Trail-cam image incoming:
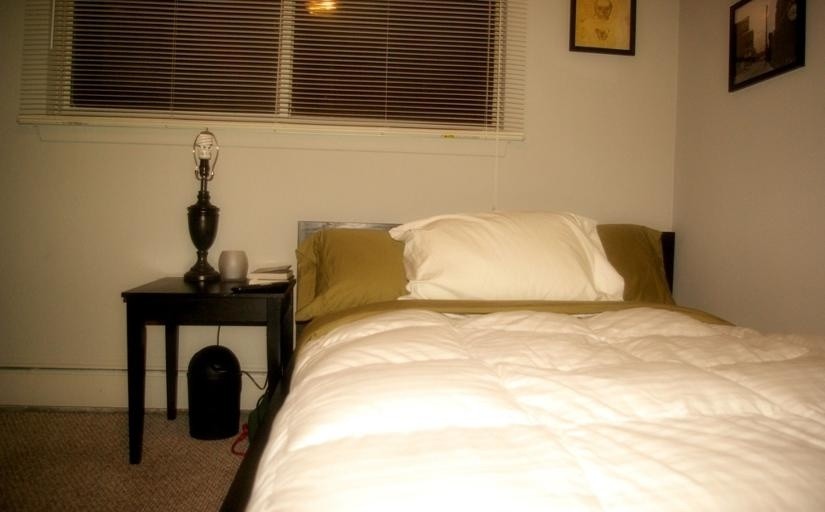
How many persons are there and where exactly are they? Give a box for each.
[579,0,629,49]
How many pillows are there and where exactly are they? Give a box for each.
[391,211,624,302]
[295,223,676,323]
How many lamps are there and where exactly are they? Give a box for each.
[181,128,221,284]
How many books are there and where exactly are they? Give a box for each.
[246,265,295,286]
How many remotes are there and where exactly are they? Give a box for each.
[231,282,290,294]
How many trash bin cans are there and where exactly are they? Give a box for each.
[186,344,243,442]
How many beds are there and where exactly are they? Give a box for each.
[219,220,825,512]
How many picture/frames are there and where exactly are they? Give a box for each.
[569,0,637,56]
[728,0,807,93]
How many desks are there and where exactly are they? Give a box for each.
[121,277,296,464]
